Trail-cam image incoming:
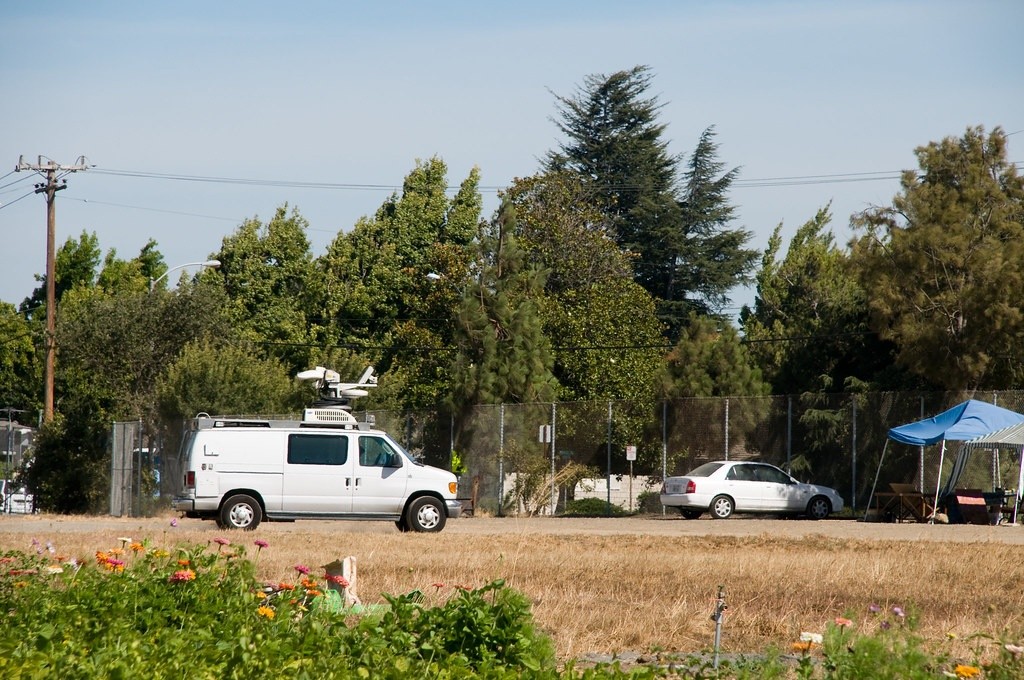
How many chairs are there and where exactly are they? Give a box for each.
[1001,495,1024,522]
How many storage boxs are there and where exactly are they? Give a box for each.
[944,489,991,524]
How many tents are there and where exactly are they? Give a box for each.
[863,400,1024,524]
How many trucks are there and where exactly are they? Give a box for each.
[1,477,36,513]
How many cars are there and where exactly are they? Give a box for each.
[659,461,845,521]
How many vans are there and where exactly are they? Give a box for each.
[172,406,461,533]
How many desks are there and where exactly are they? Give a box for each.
[874,493,936,524]
[983,492,1019,521]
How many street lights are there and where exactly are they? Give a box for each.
[134,259,222,516]
